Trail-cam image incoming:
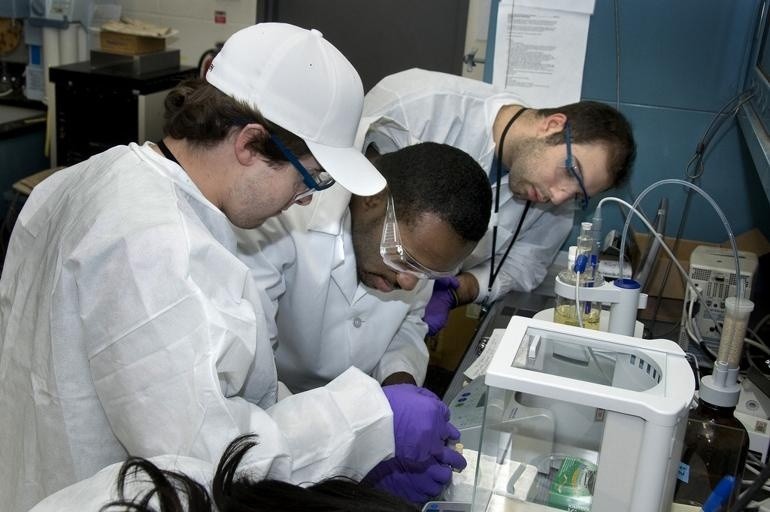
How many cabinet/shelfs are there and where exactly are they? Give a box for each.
[47,60,198,168]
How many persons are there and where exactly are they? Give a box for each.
[26,430,419,512]
[272,139,495,395]
[362,64,638,334]
[1,21,469,512]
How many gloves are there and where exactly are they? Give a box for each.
[365,429,465,503]
[380,382,454,470]
[422,277,459,334]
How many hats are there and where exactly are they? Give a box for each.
[203,19,388,198]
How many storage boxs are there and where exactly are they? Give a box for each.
[96,18,179,54]
[623,223,770,328]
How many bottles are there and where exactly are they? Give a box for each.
[672,375,750,510]
[554,221,605,330]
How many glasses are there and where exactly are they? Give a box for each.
[238,113,336,201]
[551,116,588,213]
[377,175,466,282]
